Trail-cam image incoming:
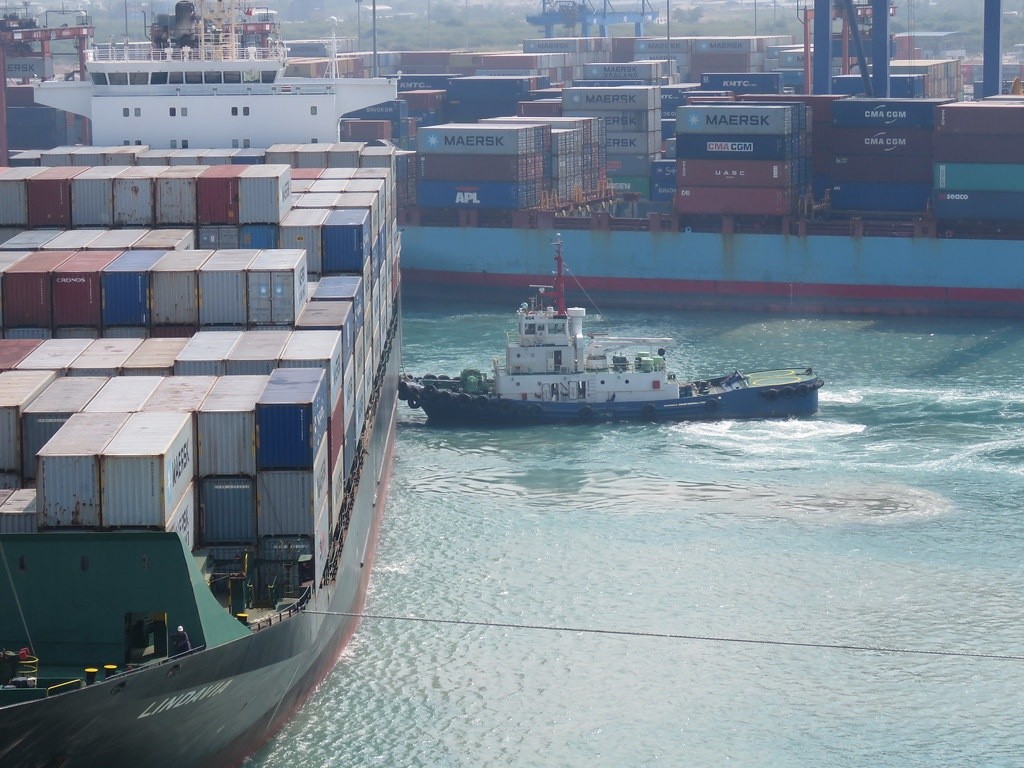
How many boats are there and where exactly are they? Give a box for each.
[397,232,823,430]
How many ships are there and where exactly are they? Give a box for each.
[0,156,397,767]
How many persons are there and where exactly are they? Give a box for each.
[172,626,191,651]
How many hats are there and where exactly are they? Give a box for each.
[177,625,183,632]
